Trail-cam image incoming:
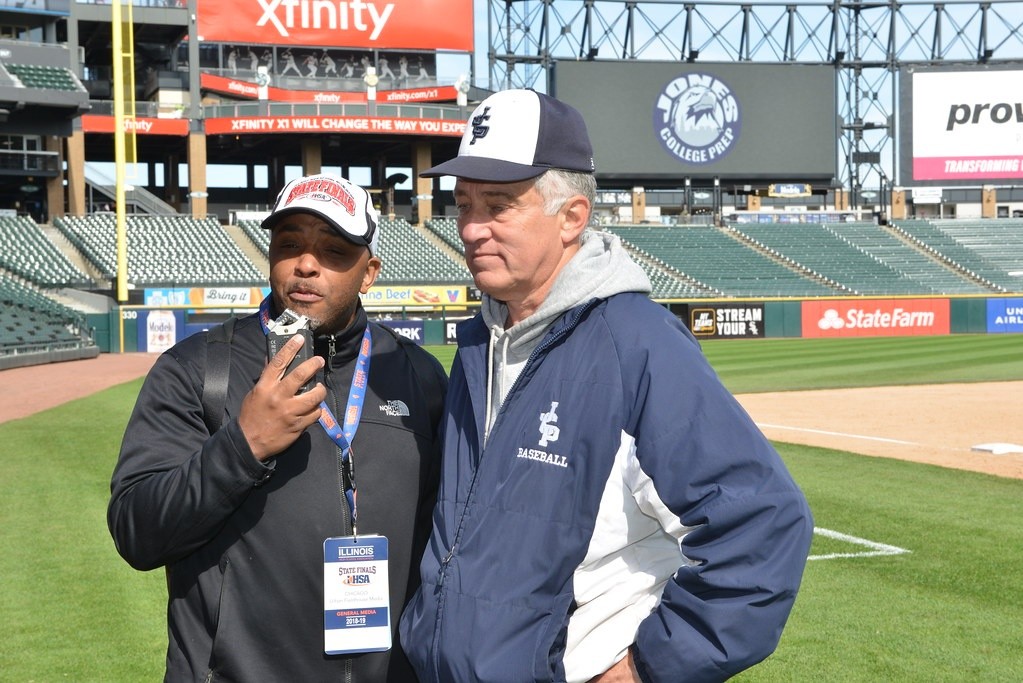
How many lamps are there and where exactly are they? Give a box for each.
[981,49,993,64]
[833,52,846,67]
[861,122,875,130]
[686,50,699,63]
[587,47,599,61]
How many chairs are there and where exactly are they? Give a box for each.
[0,61,82,92]
[0,213,476,352]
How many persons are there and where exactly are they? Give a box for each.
[227,49,429,82]
[107,176,449,683]
[397,87,814,683]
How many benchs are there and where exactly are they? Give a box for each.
[603,218,1023,299]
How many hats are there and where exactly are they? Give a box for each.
[261,173,380,257]
[418,87,597,182]
